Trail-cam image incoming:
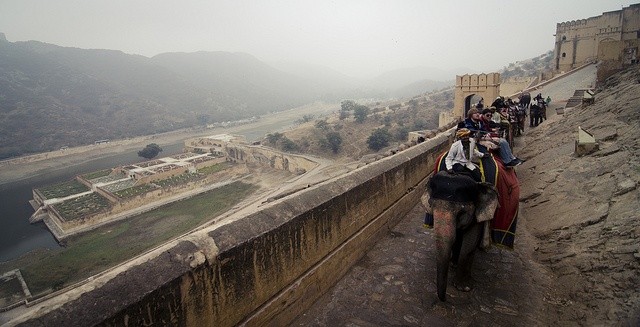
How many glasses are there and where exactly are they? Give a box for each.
[486,114,493,117]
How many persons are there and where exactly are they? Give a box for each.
[480,109,527,168]
[492,93,551,132]
[444,127,485,182]
[463,108,523,170]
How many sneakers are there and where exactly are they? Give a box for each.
[514,157,526,163]
[506,160,520,168]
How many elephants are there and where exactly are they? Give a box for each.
[529,103,543,127]
[517,92,531,109]
[420,147,520,303]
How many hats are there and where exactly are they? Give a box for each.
[456,127,471,138]
